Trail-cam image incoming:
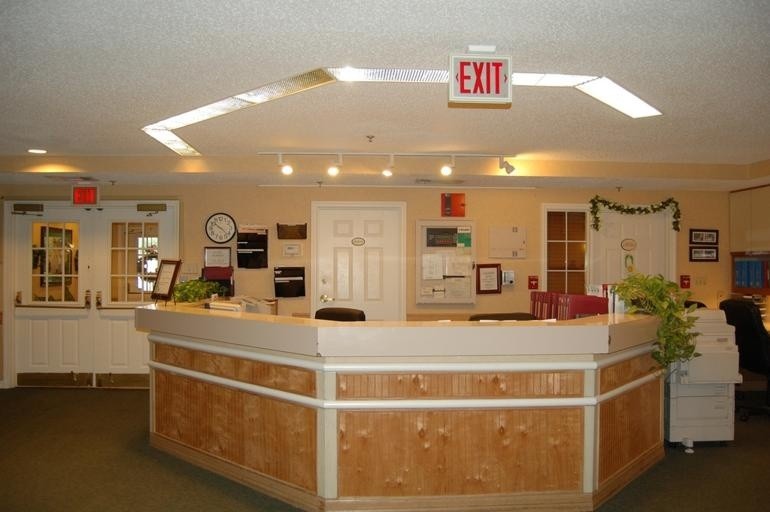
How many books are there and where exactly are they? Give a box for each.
[734,258,764,290]
[206,294,278,315]
[520,291,609,323]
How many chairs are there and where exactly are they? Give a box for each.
[720,298,770,374]
[316,307,366,320]
[469,312,537,321]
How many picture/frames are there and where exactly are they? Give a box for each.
[689,245,720,262]
[688,227,719,244]
[203,246,232,267]
[39,224,73,286]
[476,262,502,294]
[150,259,183,302]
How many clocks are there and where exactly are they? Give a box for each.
[205,212,236,244]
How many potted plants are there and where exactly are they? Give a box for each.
[610,273,702,370]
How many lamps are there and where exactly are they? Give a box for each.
[259,151,519,181]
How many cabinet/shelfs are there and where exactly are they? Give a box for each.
[663,309,743,454]
